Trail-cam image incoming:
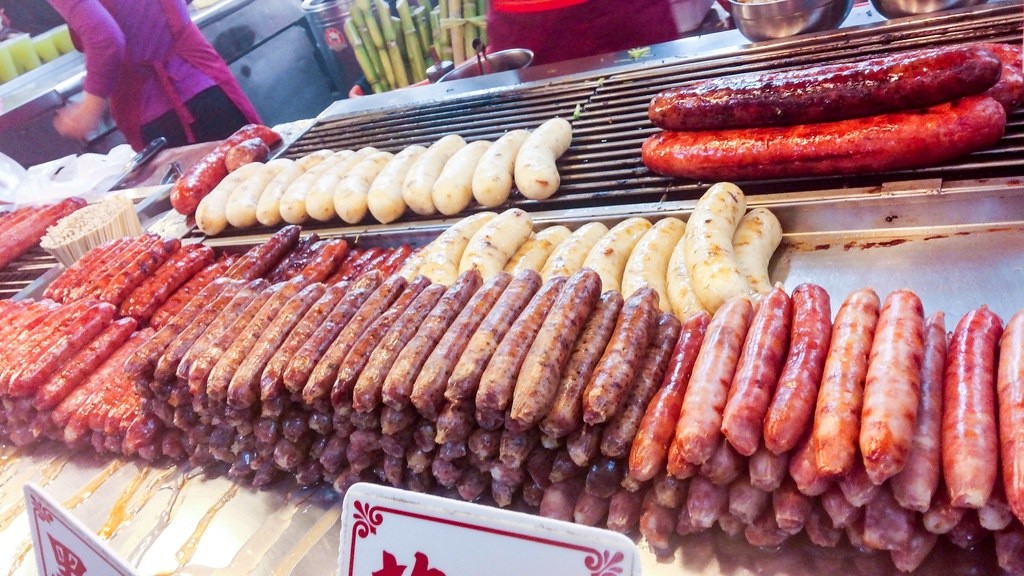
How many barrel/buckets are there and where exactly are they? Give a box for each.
[302,0,367,98]
[345,72,431,98]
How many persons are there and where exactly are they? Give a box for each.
[46,0,265,153]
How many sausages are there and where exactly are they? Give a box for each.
[0,41,1024,576]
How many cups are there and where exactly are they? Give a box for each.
[0,23,76,85]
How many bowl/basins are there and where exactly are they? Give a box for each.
[727,0,857,44]
[434,47,535,84]
[870,0,992,20]
